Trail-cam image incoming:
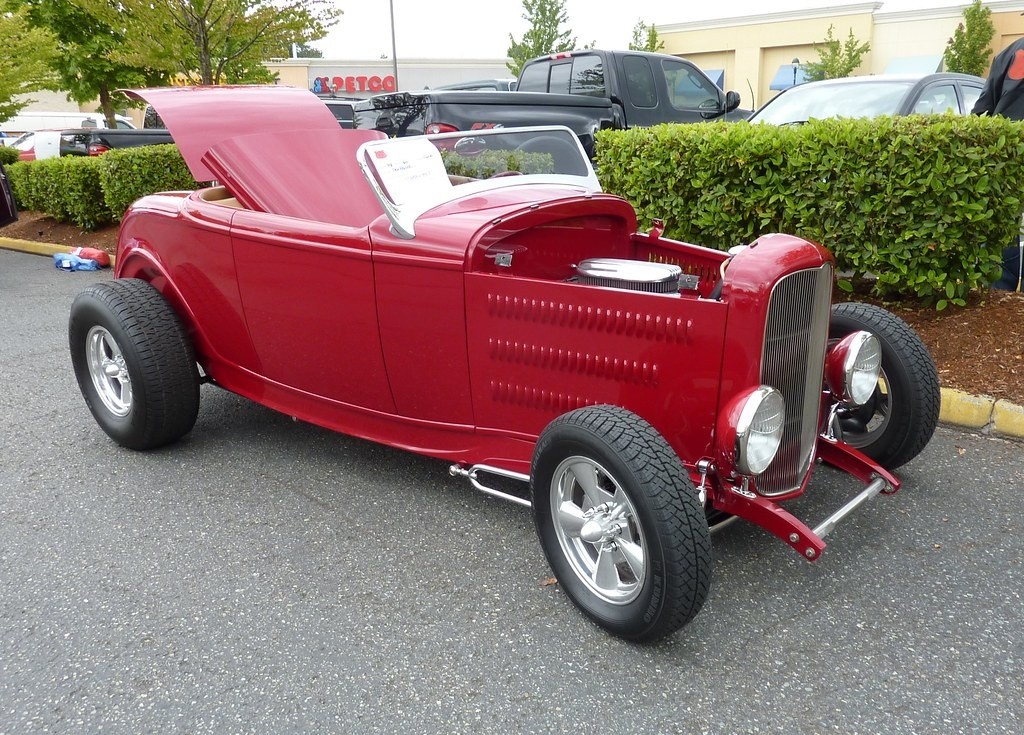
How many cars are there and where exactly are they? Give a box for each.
[10,129,60,161]
[741,72,984,125]
[65,85,942,644]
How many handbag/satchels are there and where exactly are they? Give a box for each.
[69,246,110,267]
[53,252,102,272]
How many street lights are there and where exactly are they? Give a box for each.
[791,59,799,84]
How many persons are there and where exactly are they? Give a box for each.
[970,13,1024,291]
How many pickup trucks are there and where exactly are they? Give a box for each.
[58,99,359,155]
[355,52,758,148]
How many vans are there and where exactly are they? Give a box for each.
[0,113,140,145]
